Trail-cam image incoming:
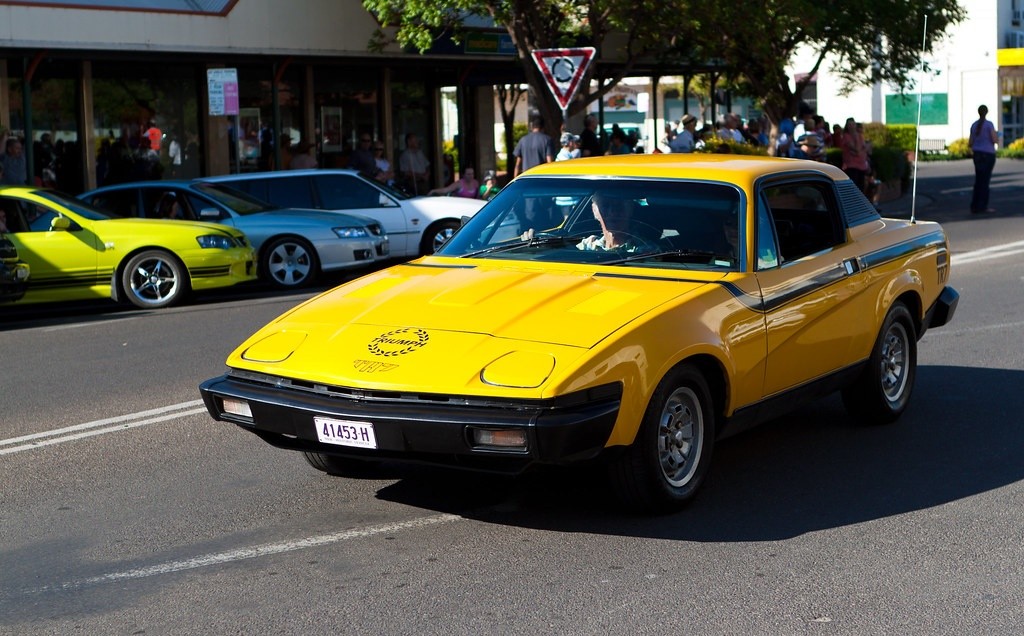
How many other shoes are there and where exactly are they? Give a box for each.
[972,208,996,216]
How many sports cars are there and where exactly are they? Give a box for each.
[200,151,961,516]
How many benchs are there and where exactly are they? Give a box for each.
[655,205,826,237]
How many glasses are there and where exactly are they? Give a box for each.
[361,140,371,143]
[373,148,383,152]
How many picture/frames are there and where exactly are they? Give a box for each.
[321,105,343,153]
[234,108,263,157]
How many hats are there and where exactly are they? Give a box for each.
[281,134,293,143]
[559,132,580,145]
[184,143,200,155]
[296,141,315,152]
[798,136,821,147]
[682,114,698,128]
[482,170,498,181]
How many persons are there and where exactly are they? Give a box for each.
[554,113,882,215]
[0,208,6,233]
[479,169,500,201]
[157,194,179,219]
[512,116,552,178]
[257,129,434,196]
[521,187,637,253]
[427,166,480,199]
[0,119,182,197]
[968,105,999,213]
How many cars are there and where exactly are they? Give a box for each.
[401,195,572,261]
[208,168,427,265]
[1,186,258,312]
[27,180,391,290]
